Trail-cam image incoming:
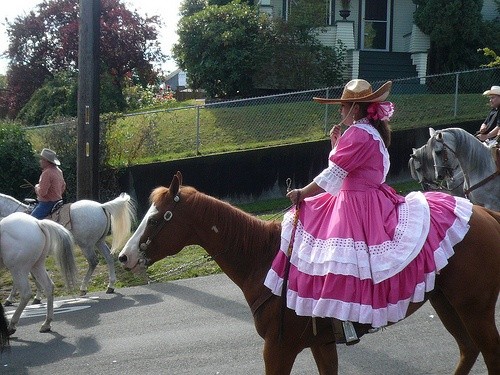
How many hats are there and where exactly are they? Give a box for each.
[483,86,500,95]
[312,78,392,126]
[33,148,61,165]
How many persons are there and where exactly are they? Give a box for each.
[263,78,474,328]
[476,86,500,149]
[28,147,67,219]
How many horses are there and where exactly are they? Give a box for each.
[119,170,500,375]
[408,127,500,211]
[0,192,136,355]
